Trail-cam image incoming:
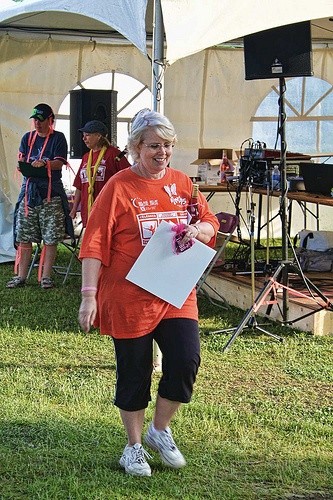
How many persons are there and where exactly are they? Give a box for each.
[6,103,76,288]
[78,109,220,477]
[68,120,131,228]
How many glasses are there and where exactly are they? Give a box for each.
[140,142,174,149]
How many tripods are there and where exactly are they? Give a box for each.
[202,77,333,354]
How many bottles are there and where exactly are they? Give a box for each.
[221,149,229,182]
[187,183,202,225]
[71,146,74,157]
[271,165,280,189]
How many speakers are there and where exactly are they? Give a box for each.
[69,89,118,160]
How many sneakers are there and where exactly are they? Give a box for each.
[145,425,186,468]
[119,441,153,477]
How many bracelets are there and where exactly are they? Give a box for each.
[191,224,200,236]
[81,287,97,292]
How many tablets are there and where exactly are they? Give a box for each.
[17,160,49,177]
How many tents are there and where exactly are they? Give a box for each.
[0,0,333,264]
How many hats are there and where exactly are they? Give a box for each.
[28,104,53,121]
[78,120,108,138]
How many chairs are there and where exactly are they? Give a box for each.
[27,196,84,284]
[196,213,238,312]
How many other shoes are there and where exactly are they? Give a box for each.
[6,278,25,288]
[42,277,53,288]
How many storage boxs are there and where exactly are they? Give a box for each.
[208,232,227,267]
[190,148,233,186]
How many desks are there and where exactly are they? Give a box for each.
[199,185,333,269]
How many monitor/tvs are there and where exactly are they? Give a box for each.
[298,161,333,197]
[243,20,314,80]
